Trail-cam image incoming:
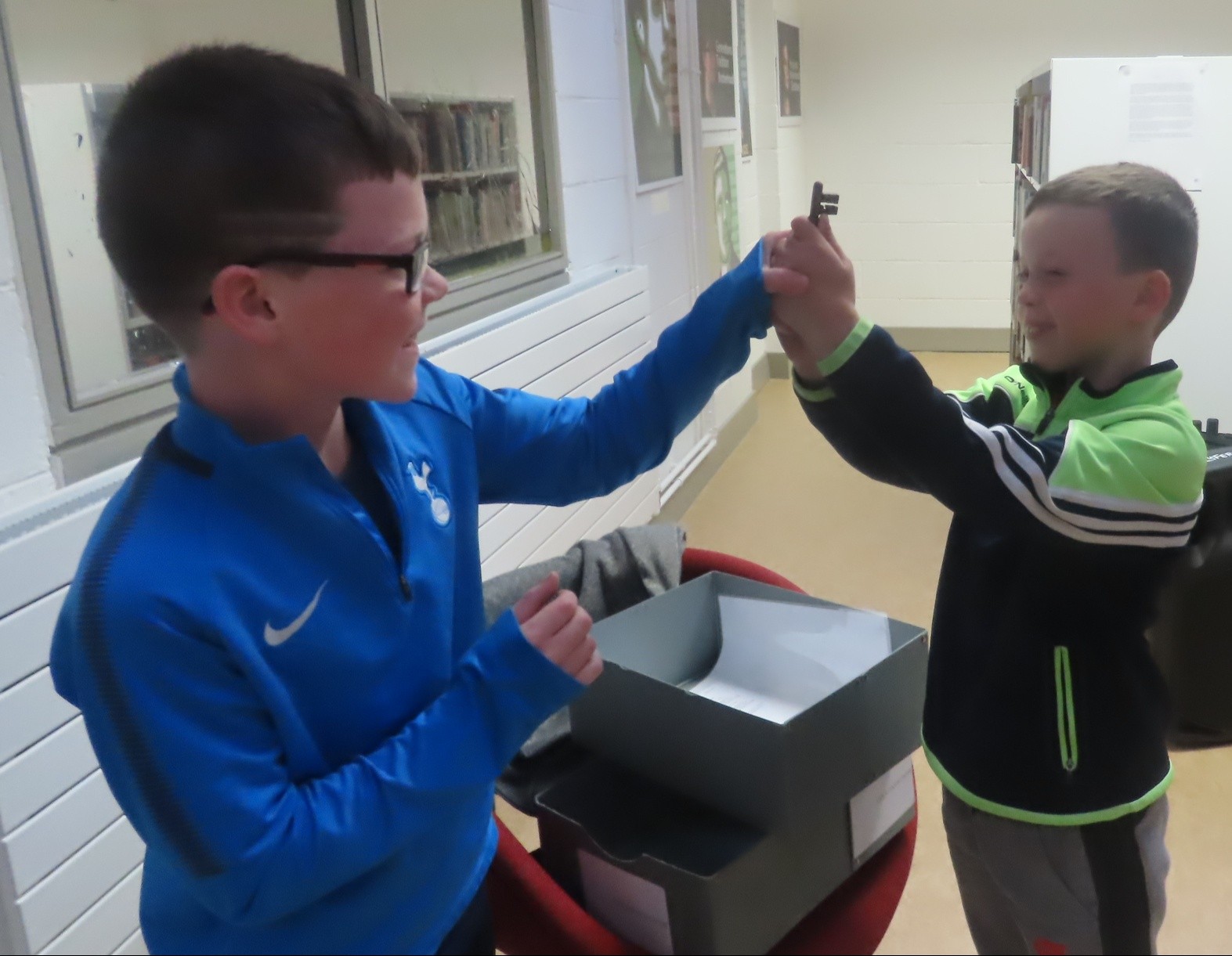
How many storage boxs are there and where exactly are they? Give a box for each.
[535,757,918,956]
[566,570,930,832]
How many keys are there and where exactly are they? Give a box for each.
[807,180,839,225]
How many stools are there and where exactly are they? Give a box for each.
[448,546,917,956]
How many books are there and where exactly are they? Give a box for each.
[1010,96,1050,366]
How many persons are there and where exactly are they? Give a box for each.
[768,163,1205,956]
[51,40,811,956]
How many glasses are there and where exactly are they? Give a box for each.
[197,239,434,317]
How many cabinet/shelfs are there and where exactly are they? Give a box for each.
[393,97,527,271]
[1010,56,1232,432]
[16,81,173,395]
